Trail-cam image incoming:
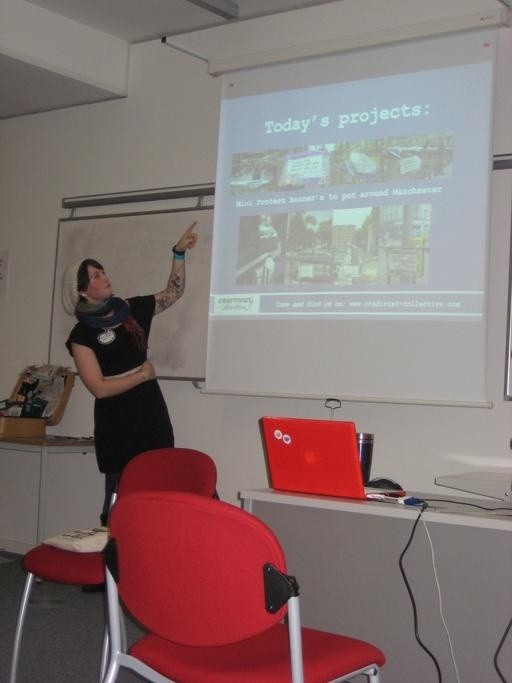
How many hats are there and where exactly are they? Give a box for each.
[60,257,94,316]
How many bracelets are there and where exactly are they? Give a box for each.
[173,253,185,260]
[173,245,185,255]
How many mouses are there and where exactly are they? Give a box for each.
[370,479,402,491]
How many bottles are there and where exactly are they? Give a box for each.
[353,431,375,486]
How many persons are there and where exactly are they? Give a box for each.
[63,221,199,593]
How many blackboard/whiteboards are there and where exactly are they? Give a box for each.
[47,205,214,381]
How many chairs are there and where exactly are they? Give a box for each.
[9,445,218,680]
[98,491,389,682]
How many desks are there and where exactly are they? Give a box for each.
[232,477,512,542]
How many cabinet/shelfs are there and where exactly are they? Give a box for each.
[1,435,100,557]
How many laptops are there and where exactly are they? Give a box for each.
[262,416,406,501]
